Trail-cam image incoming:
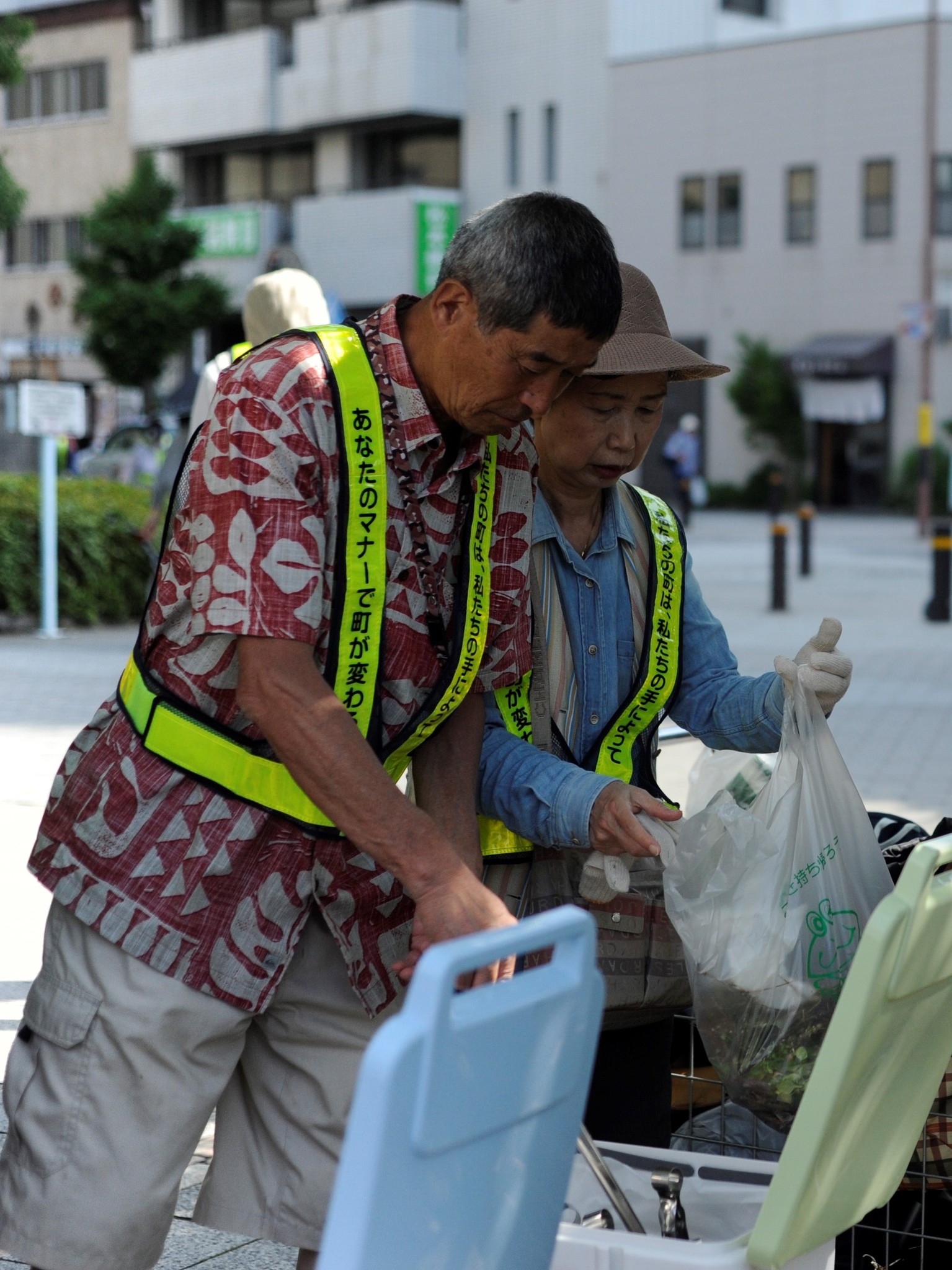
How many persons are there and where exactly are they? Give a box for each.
[659,411,701,525]
[403,263,852,1153]
[183,269,333,448]
[0,190,625,1268]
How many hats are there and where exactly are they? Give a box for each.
[568,258,731,384]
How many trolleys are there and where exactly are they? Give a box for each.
[464,822,952,1270]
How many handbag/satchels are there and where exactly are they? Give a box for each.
[633,652,895,1133]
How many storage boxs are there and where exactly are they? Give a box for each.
[549,837,952,1269]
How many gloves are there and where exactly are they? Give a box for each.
[577,801,684,907]
[772,618,854,722]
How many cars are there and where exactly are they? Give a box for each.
[69,408,185,490]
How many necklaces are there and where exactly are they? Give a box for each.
[581,492,603,557]
